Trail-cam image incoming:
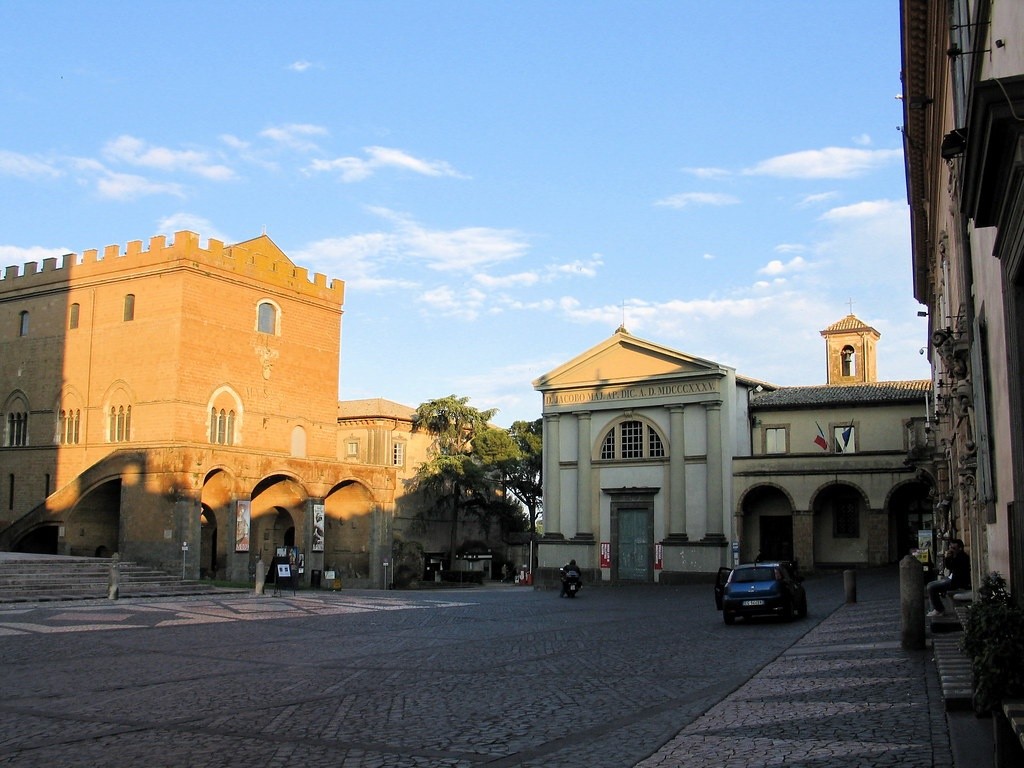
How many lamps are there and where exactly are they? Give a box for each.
[918,311,929,317]
[919,346,928,355]
[924,378,956,434]
[995,39,1004,49]
[748,384,764,393]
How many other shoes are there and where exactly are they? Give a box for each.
[924,607,934,612]
[926,609,945,616]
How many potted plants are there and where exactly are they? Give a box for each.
[959,572,1024,720]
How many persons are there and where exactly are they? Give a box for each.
[501,564,507,583]
[559,559,582,598]
[926,539,972,616]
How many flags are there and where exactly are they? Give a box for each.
[841,422,852,447]
[814,426,827,450]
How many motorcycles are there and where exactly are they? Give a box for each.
[558,567,582,599]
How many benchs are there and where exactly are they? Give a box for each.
[450,571,483,585]
[931,590,972,713]
[991,700,1024,768]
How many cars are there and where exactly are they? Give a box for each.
[714,559,808,625]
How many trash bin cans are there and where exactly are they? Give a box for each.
[311,569,322,590]
